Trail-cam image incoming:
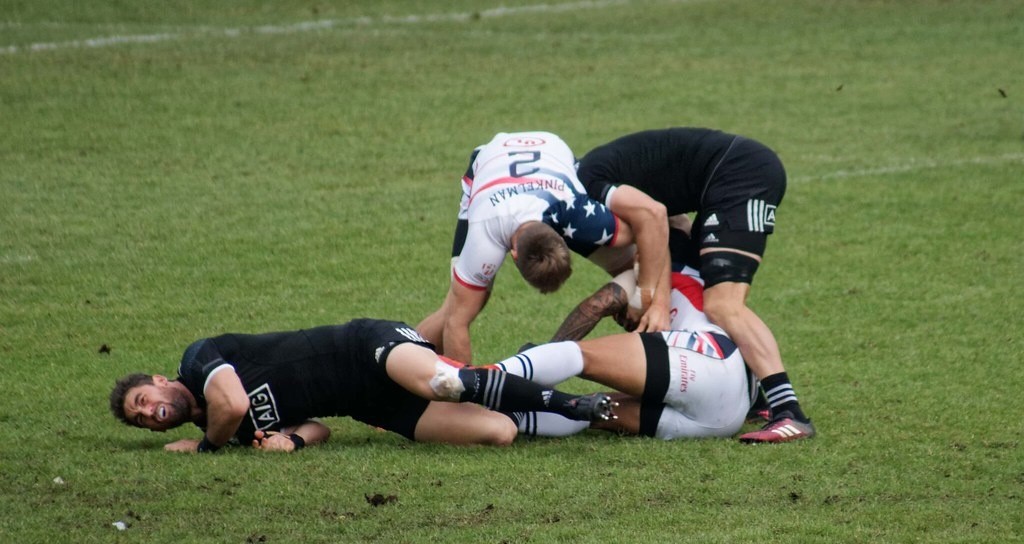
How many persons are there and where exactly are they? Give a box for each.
[110,318,619,453]
[416,128,816,444]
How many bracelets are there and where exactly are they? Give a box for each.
[197,435,218,452]
[287,433,305,452]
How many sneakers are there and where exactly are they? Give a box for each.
[739,411,816,443]
[564,393,619,422]
